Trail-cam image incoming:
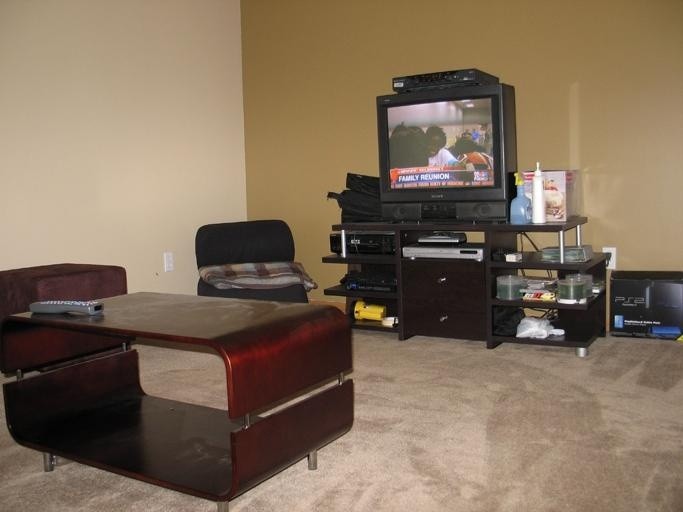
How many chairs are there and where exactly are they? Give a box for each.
[194,218,309,305]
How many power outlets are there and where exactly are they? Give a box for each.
[601,246,618,271]
[164,253,173,274]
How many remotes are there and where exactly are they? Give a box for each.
[28,300,105,317]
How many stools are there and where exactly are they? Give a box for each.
[0,263,129,378]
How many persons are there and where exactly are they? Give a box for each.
[389,122,487,168]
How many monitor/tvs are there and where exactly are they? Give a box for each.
[375,82,519,224]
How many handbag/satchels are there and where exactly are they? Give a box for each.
[327,173,382,223]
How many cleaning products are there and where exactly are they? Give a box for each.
[509,171,530,224]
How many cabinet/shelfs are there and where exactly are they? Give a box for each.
[321,218,612,357]
